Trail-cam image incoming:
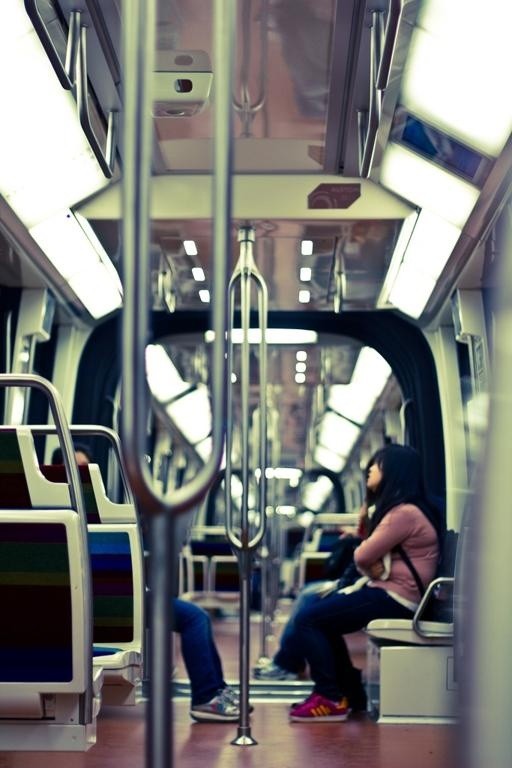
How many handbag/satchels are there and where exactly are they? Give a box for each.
[392,500,459,624]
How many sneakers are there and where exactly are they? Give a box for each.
[251,661,299,680]
[190,686,254,722]
[288,693,350,722]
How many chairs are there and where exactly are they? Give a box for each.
[0,425,146,705]
[0,372,105,754]
[297,517,466,727]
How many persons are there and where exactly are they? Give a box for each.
[252,444,446,723]
[52,445,255,721]
